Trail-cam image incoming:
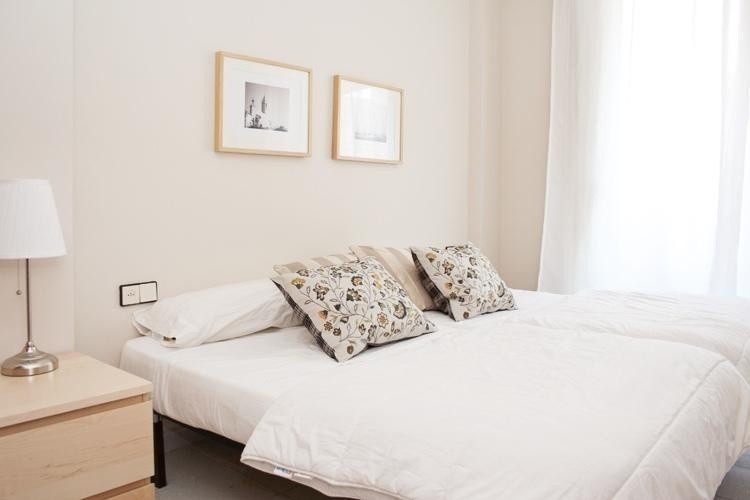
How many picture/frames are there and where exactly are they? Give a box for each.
[332,76,404,163]
[218,53,314,159]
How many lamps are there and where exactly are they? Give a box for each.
[1,180,66,377]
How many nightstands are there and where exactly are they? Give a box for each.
[0,355,156,500]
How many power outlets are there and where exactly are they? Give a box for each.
[121,281,159,304]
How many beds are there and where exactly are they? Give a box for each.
[121,289,750,500]
